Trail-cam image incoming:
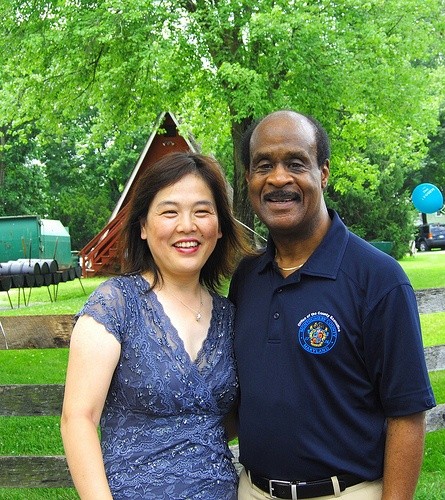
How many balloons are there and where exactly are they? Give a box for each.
[412,183,443,214]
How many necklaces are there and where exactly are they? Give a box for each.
[274,253,306,271]
[156,280,203,321]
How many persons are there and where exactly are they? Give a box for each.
[60,152,240,500]
[227,110,437,500]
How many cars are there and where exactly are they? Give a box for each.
[415,224,445,251]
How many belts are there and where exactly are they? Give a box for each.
[247,470,367,499]
